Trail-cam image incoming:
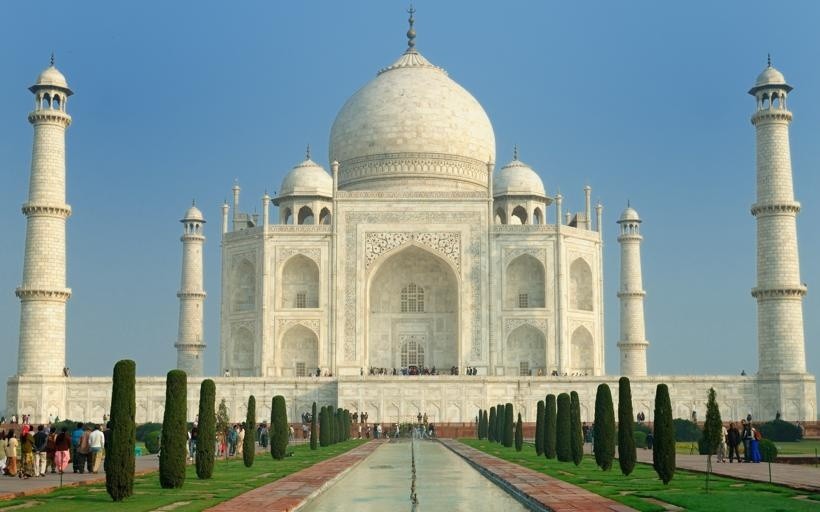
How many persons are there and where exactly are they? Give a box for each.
[360,365,478,376]
[716,419,763,464]
[224,369,231,378]
[308,366,333,376]
[528,368,588,377]
[645,431,654,449]
[582,421,596,444]
[637,412,645,424]
[1,412,113,481]
[185,419,311,462]
[350,410,435,440]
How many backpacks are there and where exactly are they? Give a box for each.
[753,430,761,440]
